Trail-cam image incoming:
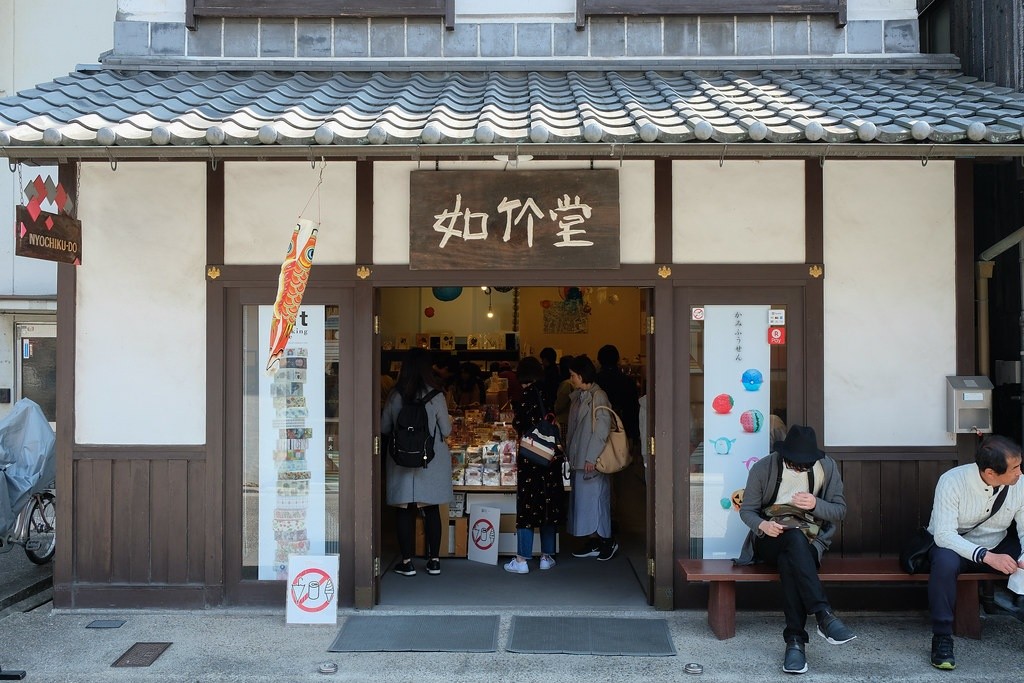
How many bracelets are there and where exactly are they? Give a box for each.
[978,549,986,563]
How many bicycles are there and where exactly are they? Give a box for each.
[0,398,56,565]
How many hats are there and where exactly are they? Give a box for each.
[773,424,825,463]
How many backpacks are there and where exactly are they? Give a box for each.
[518,382,564,468]
[387,384,441,468]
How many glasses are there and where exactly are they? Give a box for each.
[786,460,813,471]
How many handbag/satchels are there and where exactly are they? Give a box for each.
[592,389,632,474]
[752,502,826,544]
[898,520,935,574]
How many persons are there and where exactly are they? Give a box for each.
[328,346,579,421]
[924,434,1024,669]
[730,424,857,673]
[564,354,620,562]
[595,344,639,530]
[379,346,452,576]
[504,356,565,575]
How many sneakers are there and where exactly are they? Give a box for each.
[540,555,556,569]
[503,558,530,574]
[393,560,417,576]
[426,559,440,575]
[572,538,620,561]
[930,635,956,669]
[816,611,857,644]
[782,635,809,673]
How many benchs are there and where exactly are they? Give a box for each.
[678,559,1011,640]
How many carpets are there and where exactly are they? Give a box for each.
[326,612,502,653]
[507,613,681,658]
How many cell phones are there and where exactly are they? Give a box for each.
[781,522,810,529]
